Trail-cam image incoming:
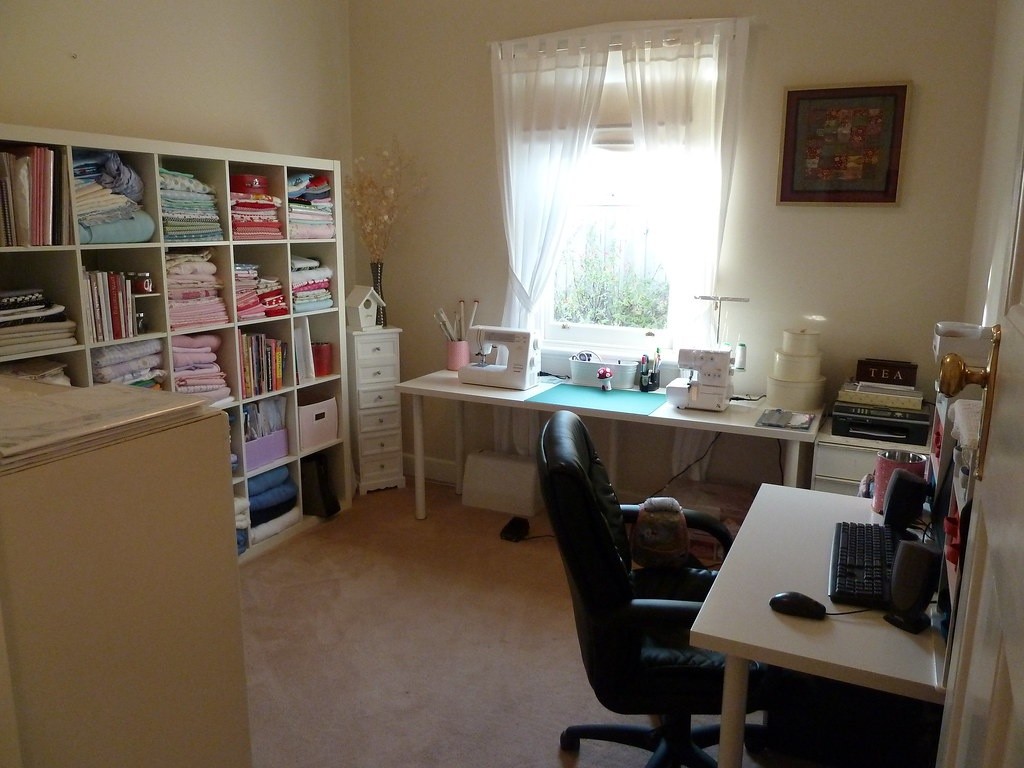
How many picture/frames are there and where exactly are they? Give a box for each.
[773,82,910,211]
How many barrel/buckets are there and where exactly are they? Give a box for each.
[872,450,927,518]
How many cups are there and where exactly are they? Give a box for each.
[640,370,649,392]
[648,370,660,391]
[447,339,470,371]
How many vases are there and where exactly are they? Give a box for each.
[368,261,387,328]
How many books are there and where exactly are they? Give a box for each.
[260,334,267,395]
[121,275,129,340]
[108,273,123,339]
[96,272,110,341]
[267,345,272,392]
[115,274,126,339]
[103,271,116,340]
[84,278,93,342]
[242,333,252,397]
[238,329,247,399]
[267,338,277,391]
[281,341,288,386]
[90,274,103,342]
[125,279,134,338]
[276,341,282,390]
[254,333,262,395]
[251,336,259,395]
[88,279,98,343]
[246,336,256,397]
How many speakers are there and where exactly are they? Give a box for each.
[883,468,928,528]
[882,540,943,634]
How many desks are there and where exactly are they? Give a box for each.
[394,364,827,523]
[686,482,944,768]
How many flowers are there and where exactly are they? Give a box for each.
[343,134,408,264]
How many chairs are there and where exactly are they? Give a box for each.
[537,410,794,768]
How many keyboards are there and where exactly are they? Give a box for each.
[828,522,897,609]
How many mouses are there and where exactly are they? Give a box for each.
[769,591,827,620]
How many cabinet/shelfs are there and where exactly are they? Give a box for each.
[811,414,932,500]
[1,123,354,565]
[346,324,407,498]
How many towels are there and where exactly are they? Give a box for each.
[0,146,344,553]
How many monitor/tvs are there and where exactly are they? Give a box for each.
[931,453,955,534]
